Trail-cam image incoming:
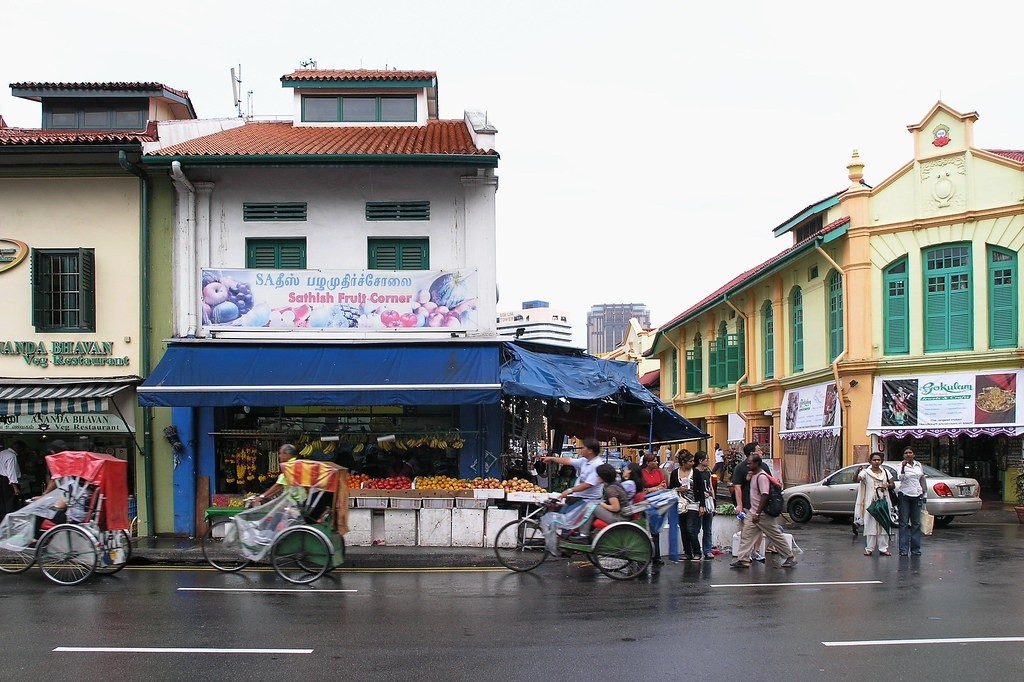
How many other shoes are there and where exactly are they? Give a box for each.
[653,559,664,565]
[705,553,714,558]
[765,544,778,553]
[900,551,908,556]
[678,554,702,561]
[911,551,921,556]
[880,551,891,556]
[864,550,871,555]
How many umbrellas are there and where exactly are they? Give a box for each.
[866,486,890,541]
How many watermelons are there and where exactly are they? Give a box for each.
[428,273,467,310]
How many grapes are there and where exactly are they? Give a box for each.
[226,281,254,316]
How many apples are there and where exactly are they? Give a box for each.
[458,476,547,492]
[348,473,371,489]
[414,475,464,490]
[201,282,239,324]
[364,476,412,489]
[411,288,462,328]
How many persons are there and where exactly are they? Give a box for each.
[248,444,307,532]
[0,440,88,547]
[891,386,914,424]
[539,437,799,568]
[853,452,896,557]
[895,447,927,556]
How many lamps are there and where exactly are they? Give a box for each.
[516,328,525,339]
[850,380,858,387]
[209,326,466,339]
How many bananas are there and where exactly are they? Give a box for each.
[296,433,465,456]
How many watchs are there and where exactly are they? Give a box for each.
[259,494,267,499]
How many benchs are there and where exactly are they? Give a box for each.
[593,492,646,528]
[39,488,100,531]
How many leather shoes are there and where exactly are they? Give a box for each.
[568,532,592,544]
[781,557,798,567]
[730,560,750,568]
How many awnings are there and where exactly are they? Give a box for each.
[135,346,503,406]
[0,385,130,417]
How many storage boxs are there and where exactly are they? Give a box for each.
[343,488,566,548]
[129,498,137,536]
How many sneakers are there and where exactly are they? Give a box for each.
[750,552,766,561]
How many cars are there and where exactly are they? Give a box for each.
[782,460,984,527]
[660,461,718,479]
[510,441,624,481]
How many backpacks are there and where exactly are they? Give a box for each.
[757,473,784,517]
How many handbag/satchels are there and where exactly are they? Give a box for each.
[673,487,688,514]
[909,502,934,535]
[883,468,899,507]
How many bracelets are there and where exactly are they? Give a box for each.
[568,488,573,494]
[657,486,659,491]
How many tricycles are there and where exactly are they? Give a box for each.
[0,452,132,585]
[200,458,346,583]
[493,487,678,581]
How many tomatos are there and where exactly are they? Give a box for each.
[381,310,416,328]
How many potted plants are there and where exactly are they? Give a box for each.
[1010,468,1024,523]
